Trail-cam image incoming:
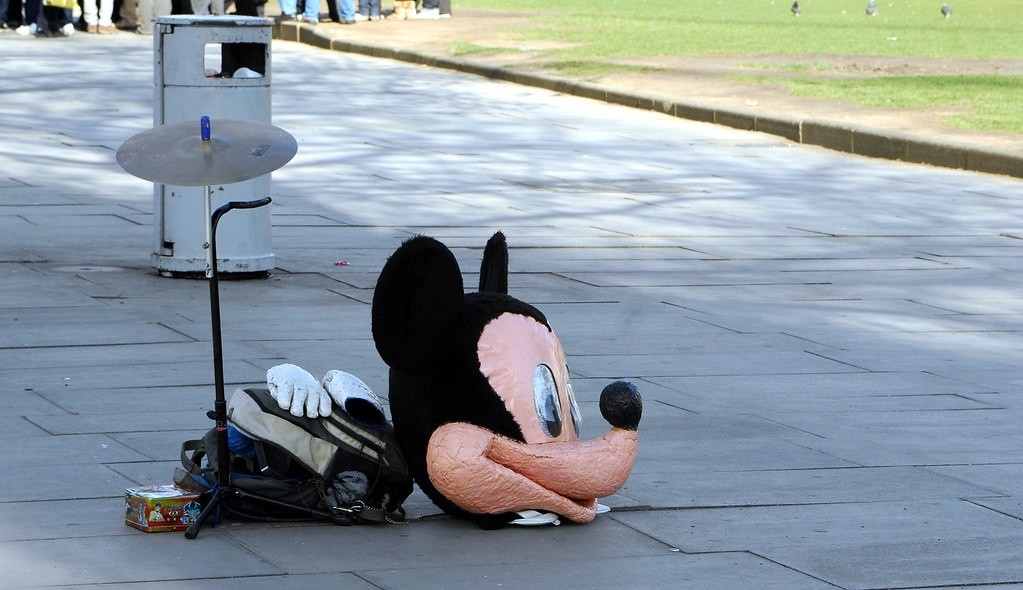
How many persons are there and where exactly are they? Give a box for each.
[0,0,452,37]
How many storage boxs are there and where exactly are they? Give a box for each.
[124,484,202,533]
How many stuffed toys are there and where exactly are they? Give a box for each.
[371,232,642,528]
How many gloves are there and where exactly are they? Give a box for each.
[266,363,332,418]
[322,369,393,434]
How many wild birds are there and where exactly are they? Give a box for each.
[791,0,801,17]
[866,0,879,17]
[941,4,951,20]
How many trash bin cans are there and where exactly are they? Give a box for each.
[150,15,277,281]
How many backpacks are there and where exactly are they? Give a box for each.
[172,387,413,526]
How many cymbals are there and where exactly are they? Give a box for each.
[115,116,300,189]
